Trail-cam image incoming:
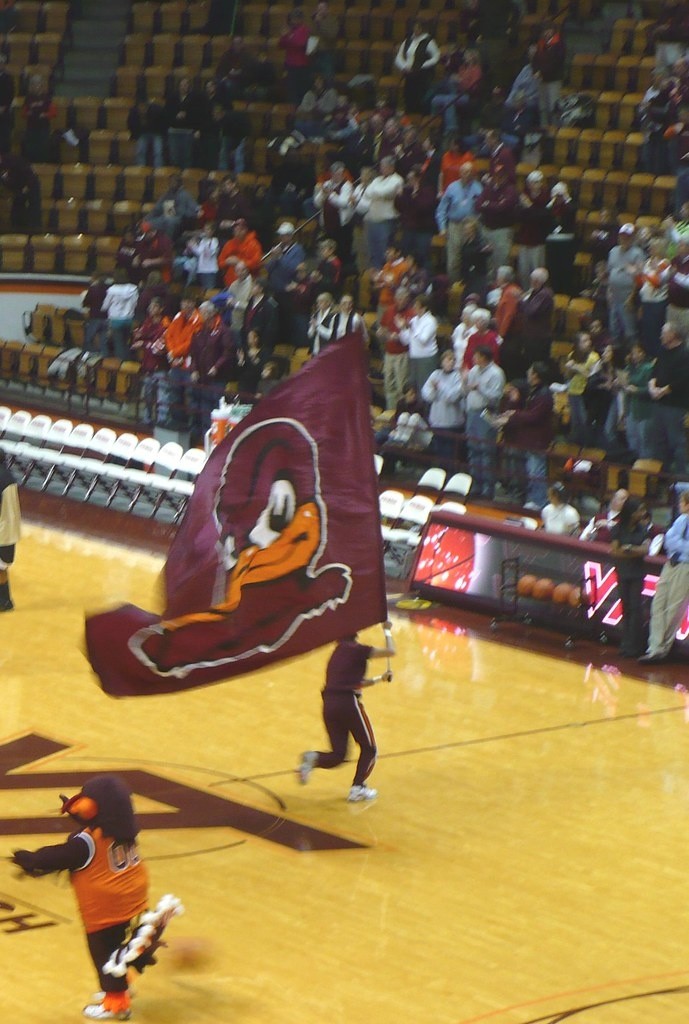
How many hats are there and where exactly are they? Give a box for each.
[527,170,543,182]
[619,222,636,234]
[277,219,295,235]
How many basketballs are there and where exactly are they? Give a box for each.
[517,574,582,609]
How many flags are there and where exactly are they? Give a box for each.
[84,333,388,696]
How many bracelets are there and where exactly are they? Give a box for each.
[384,629,392,638]
[373,675,383,686]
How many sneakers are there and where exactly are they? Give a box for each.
[297,750,318,786]
[82,1002,132,1022]
[93,987,136,1002]
[347,783,380,803]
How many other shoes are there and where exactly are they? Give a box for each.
[618,643,672,664]
[0,600,14,612]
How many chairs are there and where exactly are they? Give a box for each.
[0,0,689,505]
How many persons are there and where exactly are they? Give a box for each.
[0,0,689,665]
[299,621,394,803]
[13,772,181,1020]
